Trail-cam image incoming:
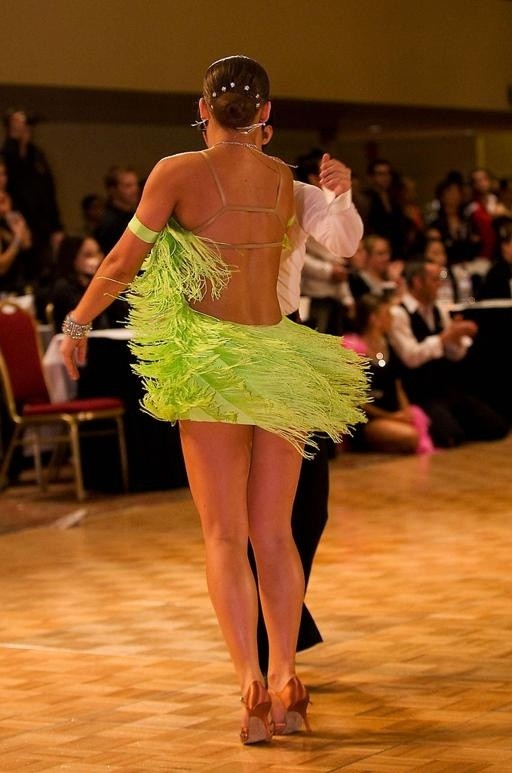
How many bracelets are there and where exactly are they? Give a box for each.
[59,318,95,341]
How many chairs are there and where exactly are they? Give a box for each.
[0,299,131,500]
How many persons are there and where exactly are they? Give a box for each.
[197,97,365,679]
[57,53,378,748]
[2,105,510,496]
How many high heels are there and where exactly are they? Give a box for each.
[239,674,313,746]
